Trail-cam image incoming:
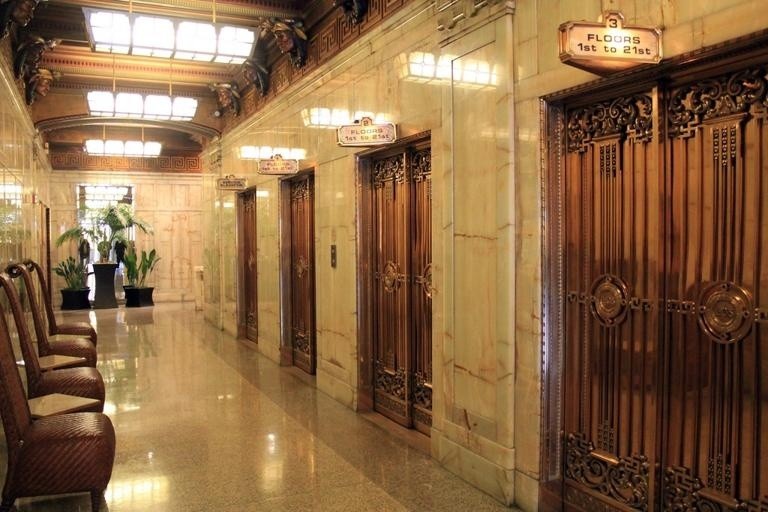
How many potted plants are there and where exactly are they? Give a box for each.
[50,201,162,309]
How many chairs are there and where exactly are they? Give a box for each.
[0,259,116,511]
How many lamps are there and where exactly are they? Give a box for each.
[236,145,306,161]
[81,78,199,123]
[394,51,523,93]
[79,1,262,68]
[81,125,161,158]
[299,107,389,131]
